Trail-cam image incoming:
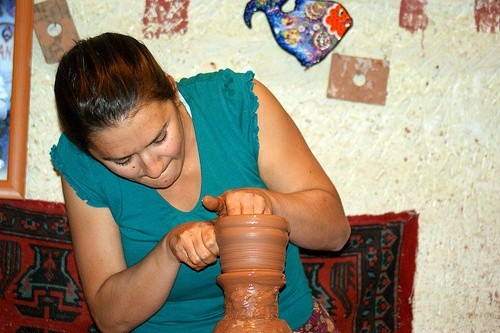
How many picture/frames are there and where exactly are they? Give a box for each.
[0,0,34,200]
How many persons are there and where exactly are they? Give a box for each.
[50,32,351,333]
